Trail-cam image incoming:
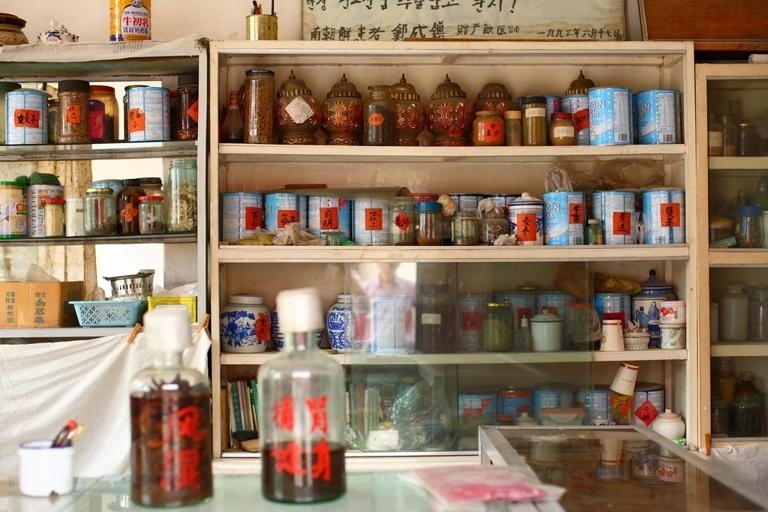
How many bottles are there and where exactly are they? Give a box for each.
[65,198,84,237]
[641,187,685,244]
[710,216,733,242]
[598,463,631,482]
[417,283,456,354]
[521,97,547,146]
[537,291,570,344]
[540,95,560,125]
[481,301,514,352]
[634,384,665,428]
[176,74,198,140]
[543,190,585,245]
[478,202,509,245]
[0,81,21,145]
[139,177,164,197]
[720,284,749,341]
[222,192,263,244]
[595,292,631,330]
[48,100,62,143]
[137,195,165,235]
[710,303,719,343]
[123,85,149,141]
[505,110,522,146]
[4,90,49,146]
[449,192,484,211]
[129,304,213,507]
[351,197,390,246]
[718,361,734,400]
[751,178,768,247]
[83,189,116,236]
[487,192,521,222]
[166,159,197,234]
[749,285,768,342]
[458,291,491,353]
[389,196,416,245]
[264,193,297,231]
[258,285,347,504]
[733,371,760,435]
[498,386,533,422]
[167,90,180,140]
[586,86,634,147]
[27,184,63,239]
[58,80,89,144]
[308,195,350,244]
[586,219,603,245]
[89,84,120,144]
[737,121,753,156]
[520,314,530,351]
[416,200,442,244]
[126,86,170,141]
[561,94,590,145]
[116,179,146,235]
[534,384,574,421]
[458,386,497,420]
[451,209,478,245]
[549,112,576,146]
[363,85,396,146]
[244,68,276,144]
[494,291,536,347]
[219,90,244,142]
[708,113,722,156]
[712,400,729,433]
[592,190,636,245]
[635,88,681,144]
[43,198,66,237]
[736,205,764,248]
[718,113,738,156]
[471,110,504,146]
[577,385,611,424]
[369,295,416,354]
[567,302,601,343]
[0,181,29,239]
[108,0,152,41]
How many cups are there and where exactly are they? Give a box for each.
[599,319,625,351]
[17,442,72,498]
[610,362,639,396]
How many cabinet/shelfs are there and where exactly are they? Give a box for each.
[693,61,765,455]
[3,450,534,508]
[0,41,208,449]
[477,425,766,512]
[209,36,699,452]
